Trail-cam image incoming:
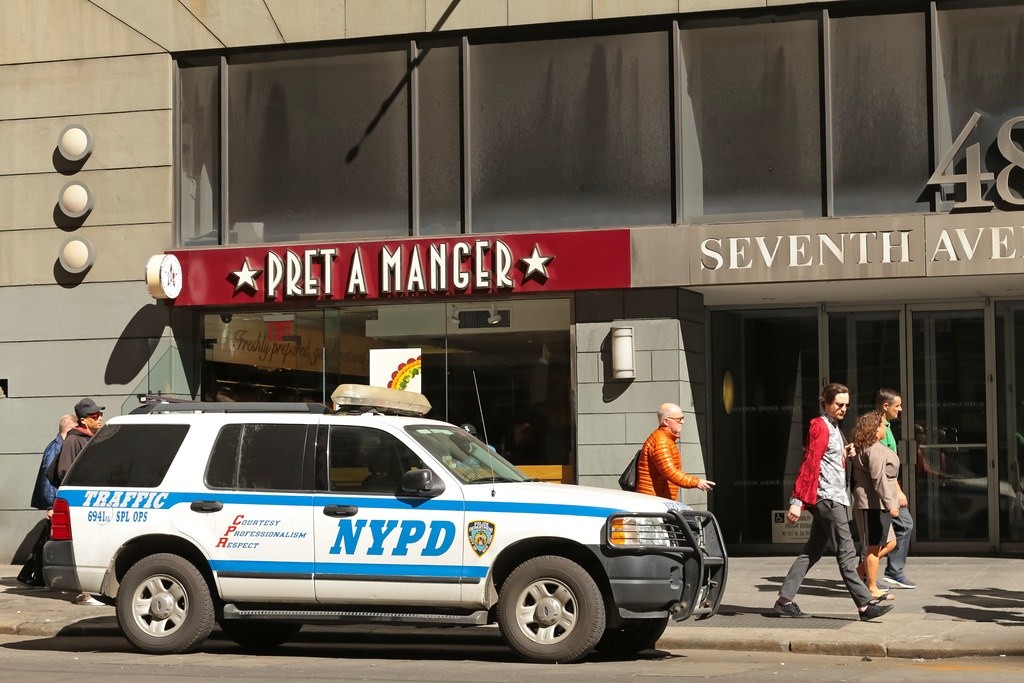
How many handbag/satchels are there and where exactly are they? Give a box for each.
[618,449,641,492]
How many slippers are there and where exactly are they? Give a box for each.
[868,592,896,601]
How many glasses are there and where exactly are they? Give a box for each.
[830,400,850,408]
[667,416,685,423]
[82,412,103,421]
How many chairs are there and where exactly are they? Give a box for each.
[363,445,411,493]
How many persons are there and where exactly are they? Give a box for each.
[851,387,916,600]
[773,382,895,622]
[912,423,958,478]
[635,402,716,501]
[459,424,496,464]
[508,422,536,465]
[17,398,105,586]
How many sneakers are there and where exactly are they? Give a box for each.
[773,600,811,618]
[863,574,890,591]
[883,576,916,588]
[859,601,894,620]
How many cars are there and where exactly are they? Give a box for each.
[720,402,1023,541]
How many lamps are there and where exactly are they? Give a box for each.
[488,303,503,325]
[446,305,461,325]
[611,325,636,379]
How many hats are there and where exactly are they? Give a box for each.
[75,398,105,419]
[460,423,477,435]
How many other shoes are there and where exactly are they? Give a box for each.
[17,574,47,588]
[76,597,106,605]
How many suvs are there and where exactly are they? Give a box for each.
[39,384,728,666]
